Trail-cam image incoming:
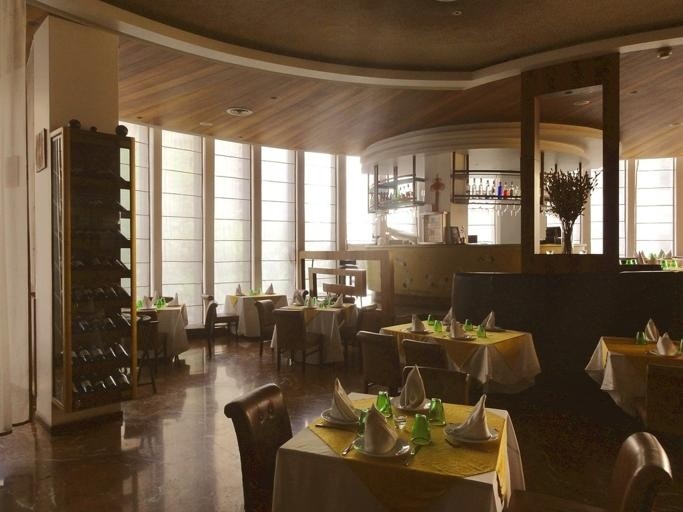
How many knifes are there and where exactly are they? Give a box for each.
[404,444,420,465]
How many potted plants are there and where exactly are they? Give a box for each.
[539,169,603,253]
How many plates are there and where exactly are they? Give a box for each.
[407,327,429,333]
[388,394,433,411]
[352,435,413,458]
[447,332,470,340]
[441,423,500,444]
[320,407,367,426]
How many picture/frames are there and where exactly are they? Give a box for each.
[444,226,462,244]
[419,210,447,244]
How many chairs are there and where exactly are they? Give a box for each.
[339,306,367,361]
[401,365,471,407]
[253,299,277,357]
[223,381,294,512]
[200,293,240,343]
[272,309,325,371]
[402,338,447,369]
[506,430,676,512]
[293,288,309,303]
[632,359,682,430]
[358,329,402,397]
[138,309,170,363]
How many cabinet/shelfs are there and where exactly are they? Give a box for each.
[51,126,137,415]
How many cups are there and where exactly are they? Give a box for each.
[412,413,431,446]
[434,320,442,333]
[154,296,168,312]
[377,391,393,418]
[477,324,486,339]
[428,315,434,326]
[428,396,445,426]
[464,319,473,332]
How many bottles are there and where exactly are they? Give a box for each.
[101,340,116,362]
[378,185,418,201]
[78,369,94,393]
[503,181,509,198]
[102,364,116,389]
[492,181,497,197]
[77,343,92,364]
[90,367,106,392]
[72,254,122,272]
[69,345,81,365]
[472,178,475,198]
[510,181,514,199]
[75,310,93,336]
[113,366,131,388]
[90,342,105,363]
[102,310,117,330]
[112,337,129,360]
[115,311,131,328]
[465,178,470,199]
[498,183,503,198]
[479,178,483,197]
[486,180,490,199]
[73,284,120,301]
